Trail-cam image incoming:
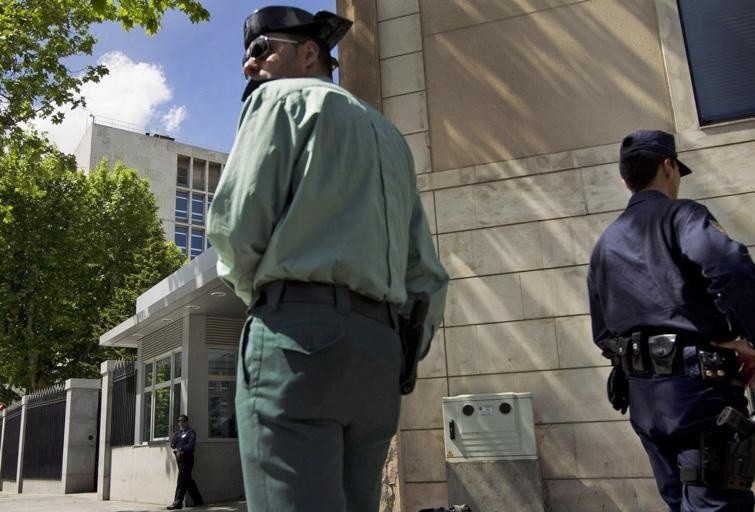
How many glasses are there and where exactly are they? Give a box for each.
[242,34,305,68]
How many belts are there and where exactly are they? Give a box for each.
[256,282,394,327]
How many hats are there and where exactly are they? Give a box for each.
[242,5,322,51]
[619,129,692,177]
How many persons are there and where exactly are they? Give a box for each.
[204,6,448,511]
[167,415,203,510]
[588,131,754,511]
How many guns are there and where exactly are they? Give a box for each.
[400,292,429,395]
[716,406,754,481]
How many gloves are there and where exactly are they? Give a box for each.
[606,367,632,415]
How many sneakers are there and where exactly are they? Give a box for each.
[166,504,183,510]
